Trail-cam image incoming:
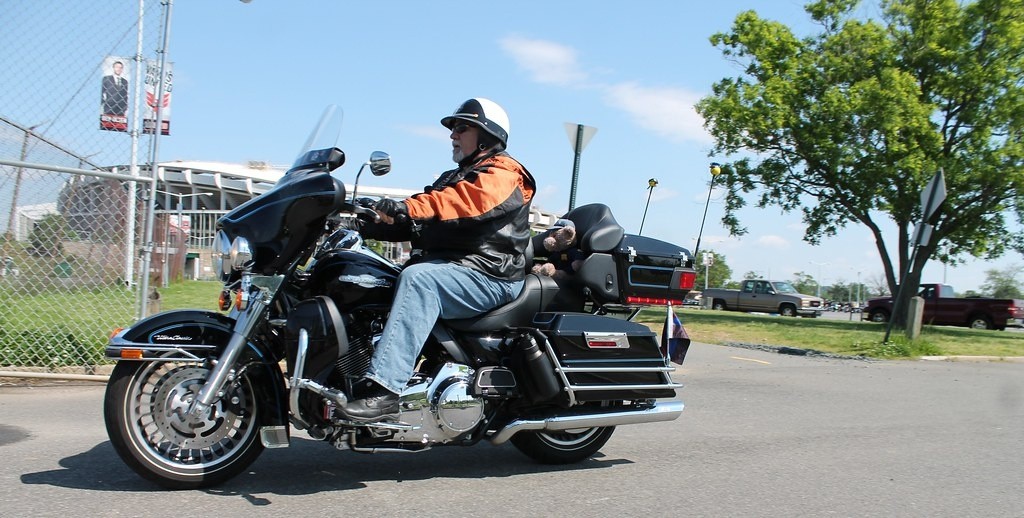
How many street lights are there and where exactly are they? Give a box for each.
[638,178,660,236]
[694,164,721,256]
[146,189,213,231]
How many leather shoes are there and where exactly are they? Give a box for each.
[336,390,401,423]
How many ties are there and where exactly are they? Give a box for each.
[117,77,119,86]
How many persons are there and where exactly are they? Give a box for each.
[101,61,128,116]
[334,97,536,425]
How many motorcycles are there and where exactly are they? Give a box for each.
[101,105,696,491]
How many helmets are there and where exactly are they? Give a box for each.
[441,98,509,149]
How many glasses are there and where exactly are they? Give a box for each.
[451,123,484,134]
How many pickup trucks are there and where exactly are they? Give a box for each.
[861,284,1024,330]
[702,279,824,318]
